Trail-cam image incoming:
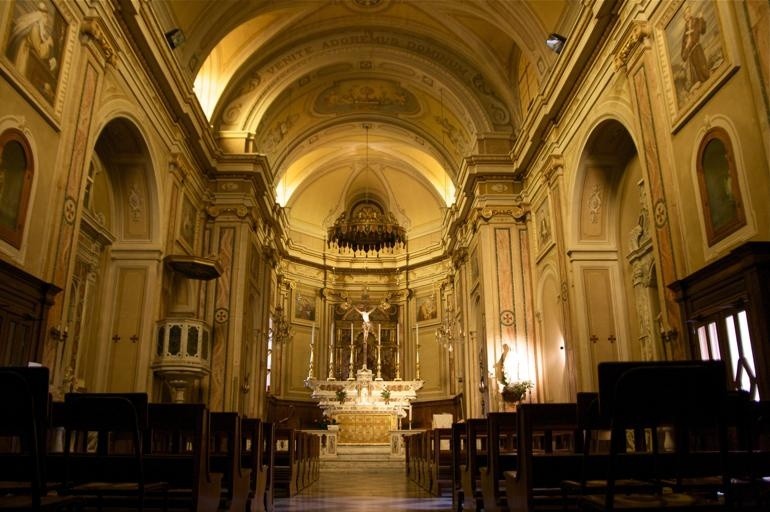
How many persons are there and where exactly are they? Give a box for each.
[352,304,379,341]
[537,209,552,249]
[6,1,58,77]
[677,5,711,95]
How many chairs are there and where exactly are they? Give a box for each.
[575,356,751,510]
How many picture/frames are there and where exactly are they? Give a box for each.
[1,0,83,133]
[652,0,745,133]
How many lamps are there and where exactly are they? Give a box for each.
[327,123,407,260]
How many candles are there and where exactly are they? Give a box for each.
[310,322,353,346]
[378,322,419,346]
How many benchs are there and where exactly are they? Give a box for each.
[1,365,320,511]
[402,402,769,511]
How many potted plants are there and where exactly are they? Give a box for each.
[381,390,391,404]
[336,391,346,405]
